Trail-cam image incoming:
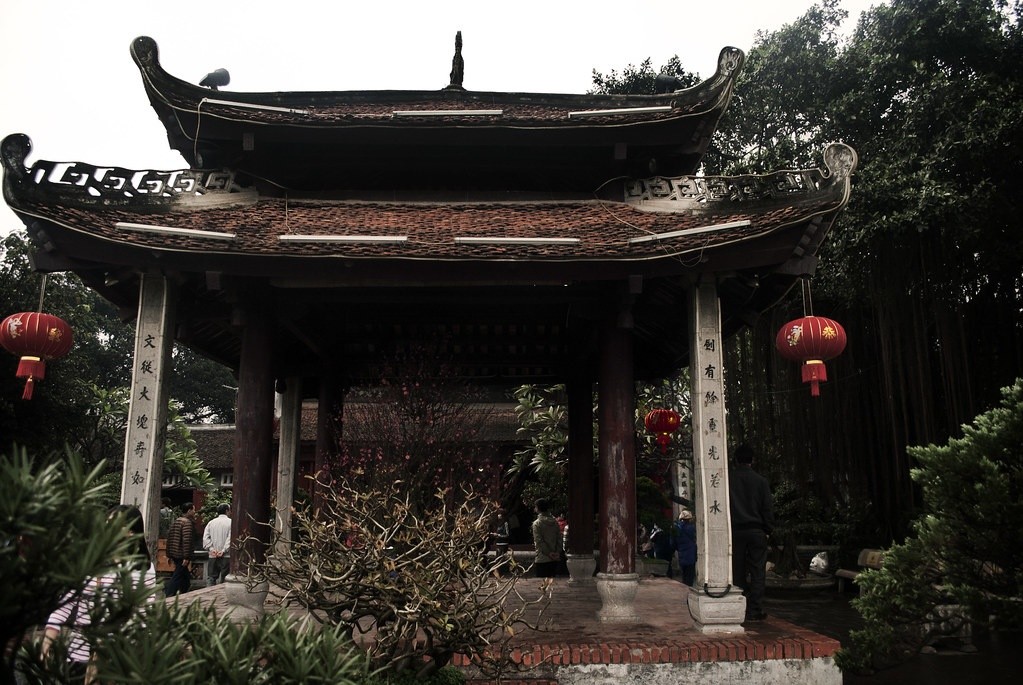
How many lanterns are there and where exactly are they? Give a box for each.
[776,315,846,396]
[0,311,73,399]
[644,409,680,452]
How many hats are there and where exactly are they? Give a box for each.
[679,511,692,520]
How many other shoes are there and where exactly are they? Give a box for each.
[746,610,767,622]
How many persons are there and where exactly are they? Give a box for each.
[494,510,511,575]
[39,504,157,685]
[554,508,569,575]
[637,521,673,577]
[166,502,196,598]
[728,445,774,620]
[532,498,562,577]
[161,497,172,517]
[202,503,232,587]
[669,510,697,587]
